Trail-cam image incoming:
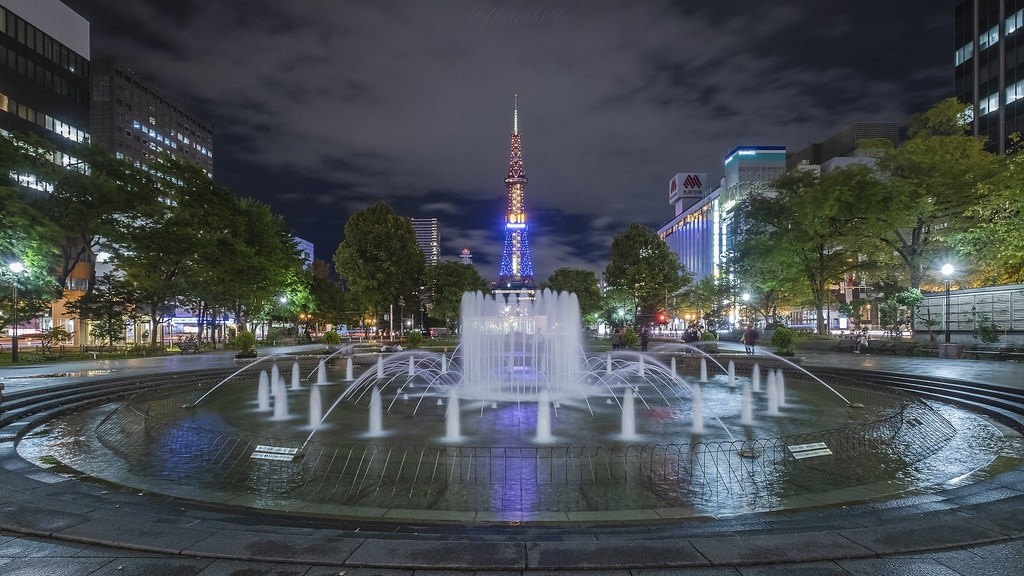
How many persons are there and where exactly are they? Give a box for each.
[641,327,650,351]
[611,326,626,351]
[686,324,717,353]
[740,325,757,355]
[853,327,869,353]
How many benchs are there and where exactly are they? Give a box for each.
[175,342,200,355]
[961,343,1014,362]
[834,340,919,356]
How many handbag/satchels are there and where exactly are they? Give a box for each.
[681,334,686,340]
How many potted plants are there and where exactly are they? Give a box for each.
[321,331,341,355]
[770,327,794,353]
[229,329,258,367]
[696,332,719,353]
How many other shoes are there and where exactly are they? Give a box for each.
[853,350,860,353]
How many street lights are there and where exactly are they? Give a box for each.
[399,295,406,337]
[10,262,24,363]
